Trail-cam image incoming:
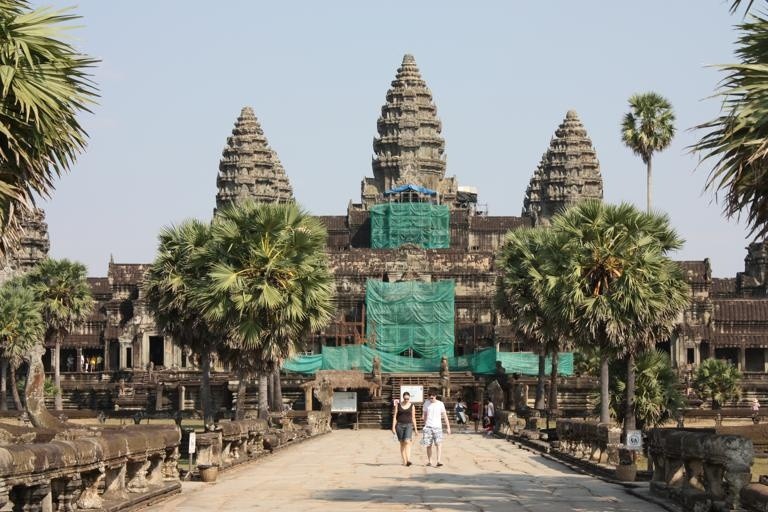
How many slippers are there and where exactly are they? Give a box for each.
[436,464,443,466]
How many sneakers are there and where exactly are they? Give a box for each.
[407,461,411,465]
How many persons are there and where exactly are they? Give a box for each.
[392,391,420,467]
[66,352,105,372]
[485,397,495,431]
[454,397,468,432]
[752,397,761,412]
[469,398,482,433]
[421,391,451,467]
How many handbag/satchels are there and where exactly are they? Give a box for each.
[457,407,463,411]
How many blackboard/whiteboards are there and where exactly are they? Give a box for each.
[399,384,424,405]
[331,391,358,414]
